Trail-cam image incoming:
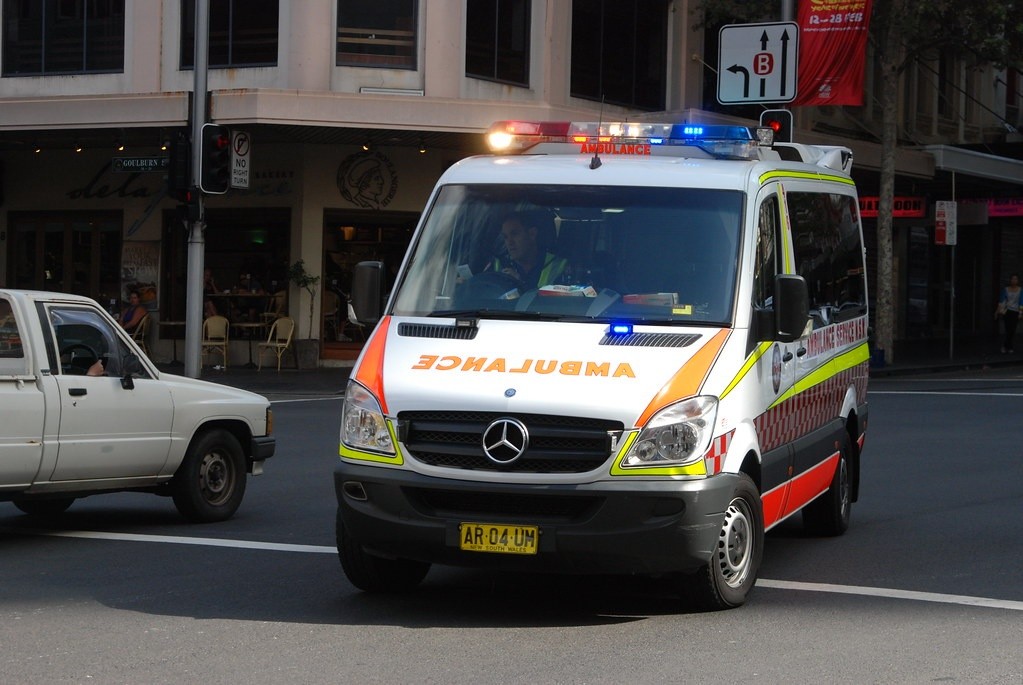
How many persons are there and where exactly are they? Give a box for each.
[55,325,104,376]
[334,259,352,342]
[456,212,589,294]
[999,274,1023,354]
[203,269,222,317]
[274,283,289,313]
[119,289,146,340]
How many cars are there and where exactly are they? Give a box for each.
[0,288,277,522]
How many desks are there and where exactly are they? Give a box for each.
[259,313,287,338]
[324,316,340,340]
[204,291,270,338]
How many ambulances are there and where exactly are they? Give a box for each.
[335,121,872,616]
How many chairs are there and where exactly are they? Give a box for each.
[131,312,155,366]
[257,316,298,372]
[202,315,230,372]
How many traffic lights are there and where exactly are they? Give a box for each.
[197,122,235,197]
[182,187,200,224]
[759,109,796,146]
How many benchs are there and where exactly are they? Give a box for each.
[160,321,185,339]
[229,323,269,338]
